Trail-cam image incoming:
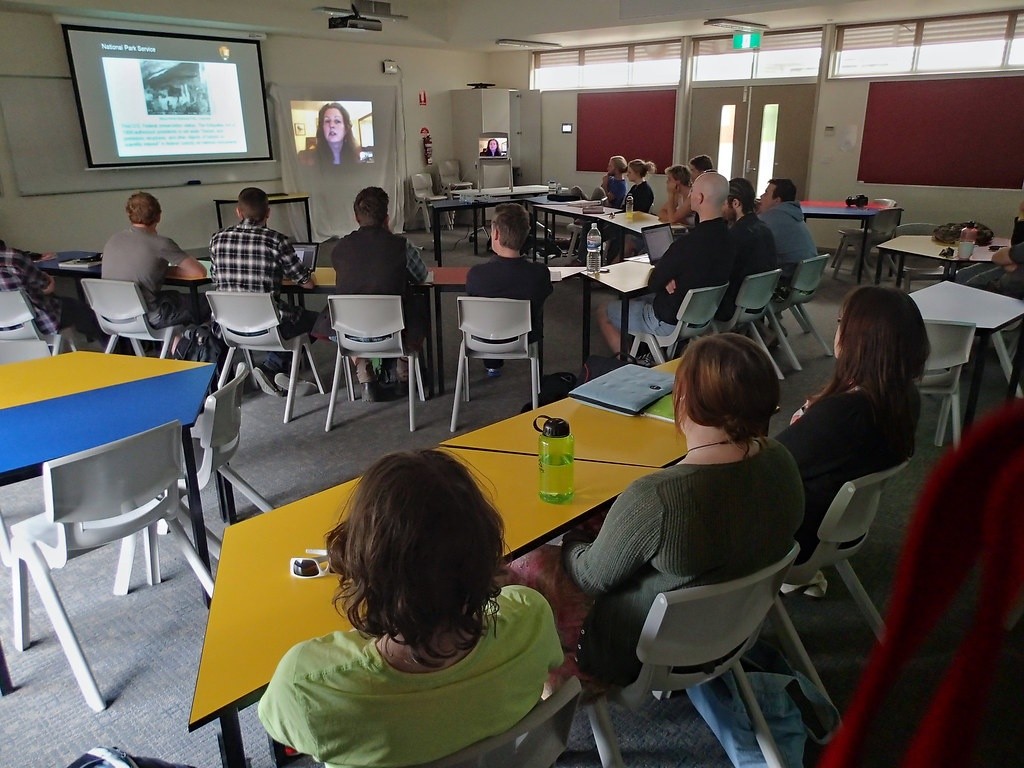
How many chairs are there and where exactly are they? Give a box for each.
[407,159,473,233]
[629,281,729,367]
[204,290,326,426]
[80,278,185,360]
[0,285,276,712]
[416,457,911,768]
[324,294,425,432]
[712,196,1024,450]
[830,200,898,281]
[447,296,541,433]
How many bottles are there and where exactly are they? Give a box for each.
[556,183,562,196]
[961,221,977,244]
[626,193,633,219]
[549,181,556,195]
[533,415,576,504]
[586,223,601,274]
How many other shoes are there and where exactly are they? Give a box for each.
[746,321,777,349]
[565,258,586,267]
[767,322,788,347]
[484,368,499,376]
[274,372,318,397]
[253,364,284,398]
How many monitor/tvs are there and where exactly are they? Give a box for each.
[478,133,509,160]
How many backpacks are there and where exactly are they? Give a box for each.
[174,324,235,397]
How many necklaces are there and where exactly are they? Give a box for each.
[687,438,733,452]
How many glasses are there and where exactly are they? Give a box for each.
[290,548,338,578]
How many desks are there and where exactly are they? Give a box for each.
[187,446,667,768]
[905,280,1024,429]
[0,350,215,697]
[873,234,1012,289]
[41,181,689,399]
[798,199,905,285]
[438,356,688,468]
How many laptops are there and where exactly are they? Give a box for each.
[641,222,673,265]
[283,241,319,278]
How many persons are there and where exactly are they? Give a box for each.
[297,103,362,167]
[777,284,932,566]
[485,139,501,156]
[209,187,320,398]
[505,333,805,700]
[659,155,714,225]
[101,192,211,355]
[256,447,563,768]
[566,155,628,233]
[596,172,817,364]
[333,187,429,404]
[0,240,148,355]
[571,159,657,267]
[463,203,552,378]
[956,203,1024,301]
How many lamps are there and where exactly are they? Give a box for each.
[495,38,562,51]
[703,19,770,32]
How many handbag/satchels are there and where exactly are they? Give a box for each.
[567,364,675,416]
[583,206,605,214]
[68,746,137,768]
[686,657,842,768]
[576,352,637,388]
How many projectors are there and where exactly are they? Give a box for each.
[328,15,383,33]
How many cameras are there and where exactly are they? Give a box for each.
[846,193,869,209]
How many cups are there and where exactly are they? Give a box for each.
[958,242,978,258]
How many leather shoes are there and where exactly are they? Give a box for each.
[361,382,381,401]
[400,380,409,393]
